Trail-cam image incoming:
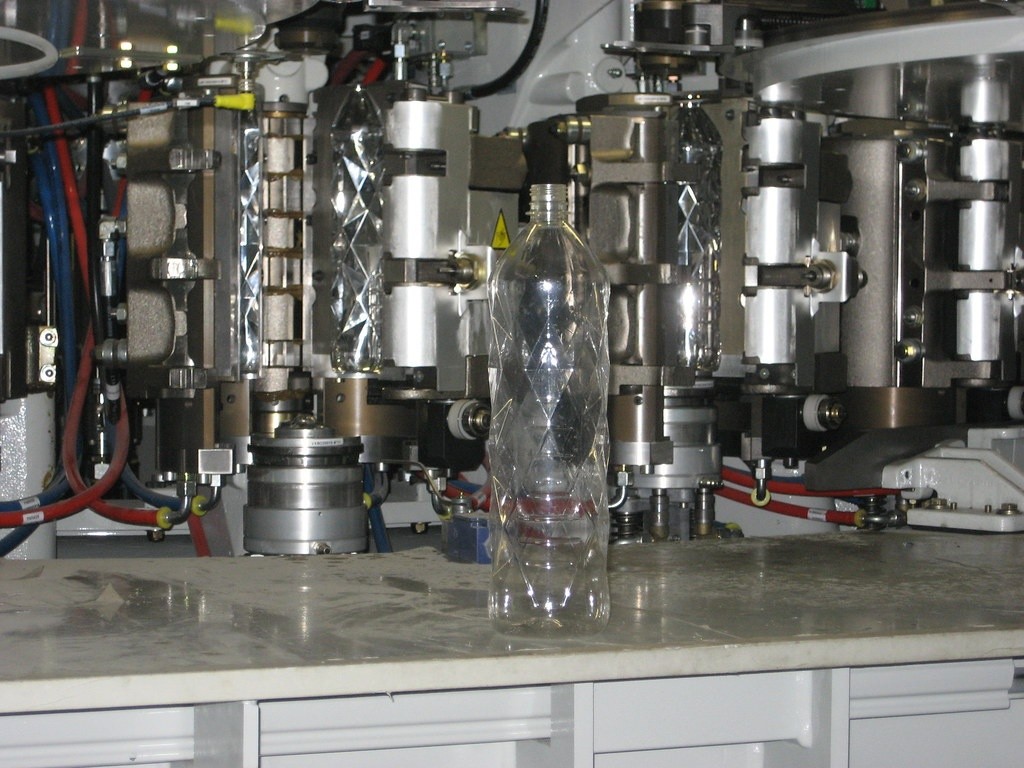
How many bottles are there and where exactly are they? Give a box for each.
[485,121,613,631]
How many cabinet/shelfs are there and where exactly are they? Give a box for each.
[0,531,1024,768]
